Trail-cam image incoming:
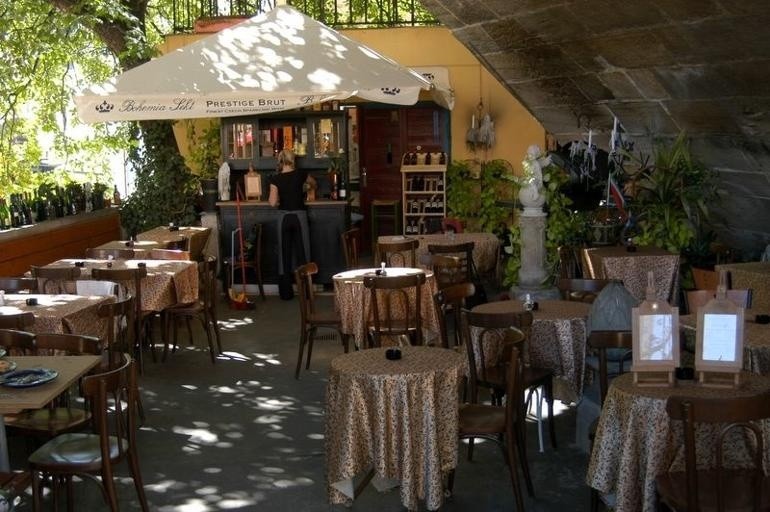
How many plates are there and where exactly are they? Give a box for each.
[0,348,17,374]
[2,367,59,388]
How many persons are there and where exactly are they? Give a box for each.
[267,147,317,302]
[517,142,544,202]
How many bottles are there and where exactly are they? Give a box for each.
[0,179,124,229]
[330,173,339,201]
[406,218,420,233]
[406,176,444,214]
[339,171,347,200]
[107,256,113,268]
[404,149,445,166]
[523,294,534,312]
[379,262,387,277]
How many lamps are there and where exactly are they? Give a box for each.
[464,62,495,148]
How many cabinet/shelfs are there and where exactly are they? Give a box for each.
[219,108,349,171]
[400,150,450,236]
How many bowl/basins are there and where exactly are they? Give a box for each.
[532,301,539,311]
[138,262,146,267]
[75,262,85,267]
[375,270,382,276]
[385,349,402,360]
[626,245,637,252]
[25,298,37,306]
